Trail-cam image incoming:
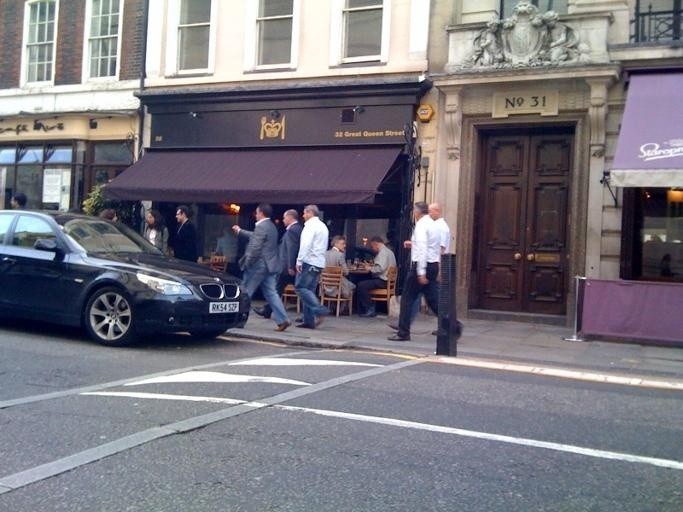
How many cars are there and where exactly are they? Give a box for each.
[0,205,251,349]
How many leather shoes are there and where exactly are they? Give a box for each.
[386,321,410,341]
[252,307,376,331]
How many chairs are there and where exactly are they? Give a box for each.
[195,255,396,318]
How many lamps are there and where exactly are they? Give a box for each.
[31,114,59,130]
[87,115,114,130]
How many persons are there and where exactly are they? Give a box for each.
[385,200,464,341]
[99,208,119,223]
[141,209,169,254]
[171,204,204,264]
[231,205,332,333]
[323,233,356,314]
[658,251,681,279]
[8,191,28,208]
[356,235,396,317]
